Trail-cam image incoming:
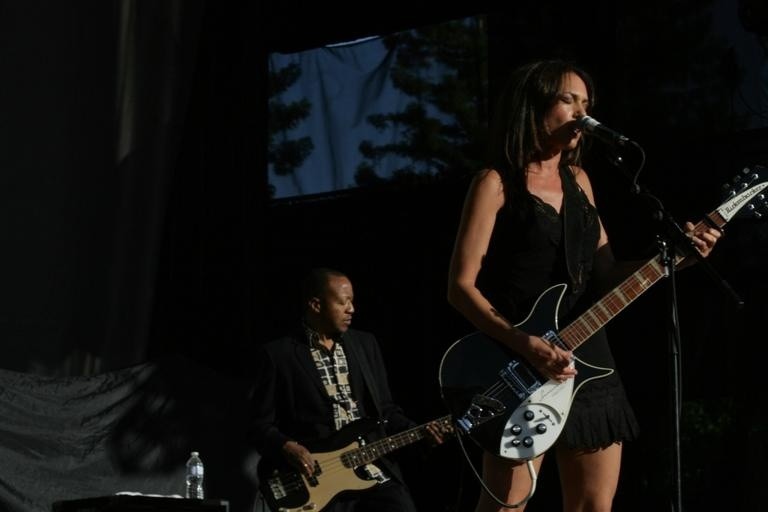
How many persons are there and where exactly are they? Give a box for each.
[244,262,455,512]
[446,59,723,512]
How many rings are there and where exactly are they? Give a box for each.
[303,464,308,468]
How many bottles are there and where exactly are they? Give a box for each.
[184,451,206,499]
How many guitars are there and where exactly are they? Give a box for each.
[438,167,767,461]
[256,414,453,511]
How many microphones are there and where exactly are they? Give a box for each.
[575,114,627,149]
